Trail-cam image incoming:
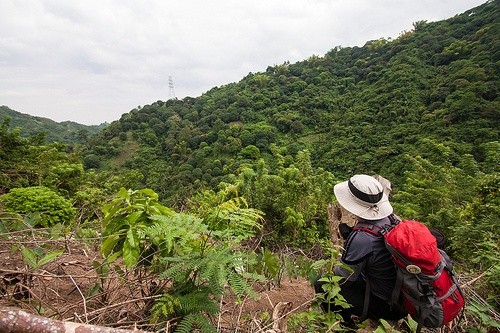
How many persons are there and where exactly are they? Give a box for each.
[313,174,409,333]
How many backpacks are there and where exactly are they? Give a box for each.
[352,212,465,333]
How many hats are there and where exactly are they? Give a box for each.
[333,174,394,220]
